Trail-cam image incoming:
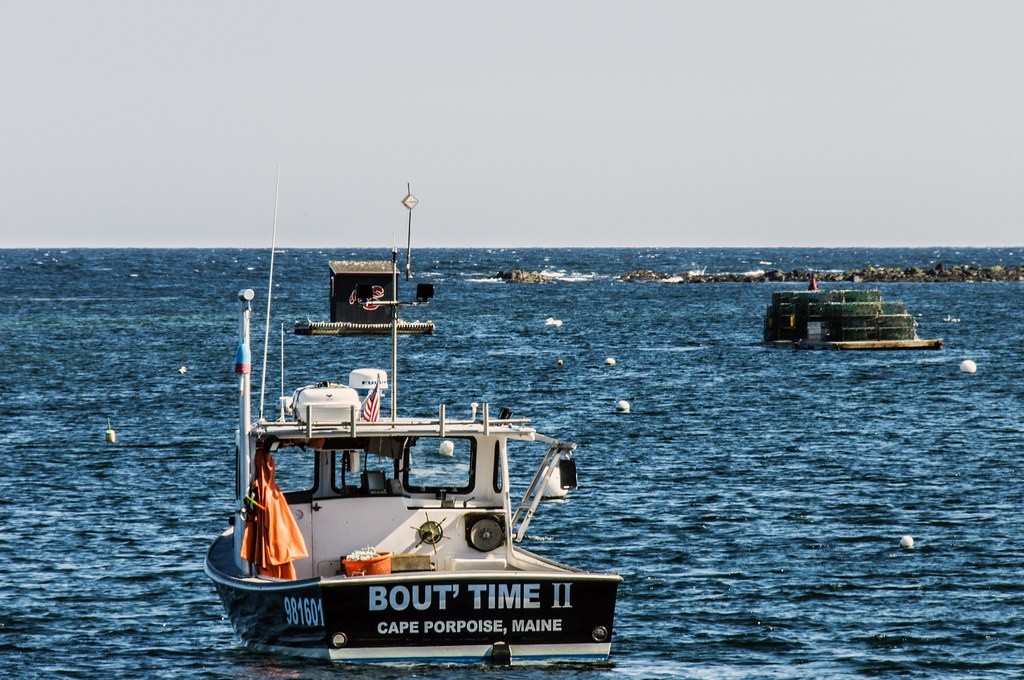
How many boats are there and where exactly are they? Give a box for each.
[762,288,944,350]
[293,259,436,335]
[203,160,625,669]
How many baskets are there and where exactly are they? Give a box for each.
[342,551,392,577]
